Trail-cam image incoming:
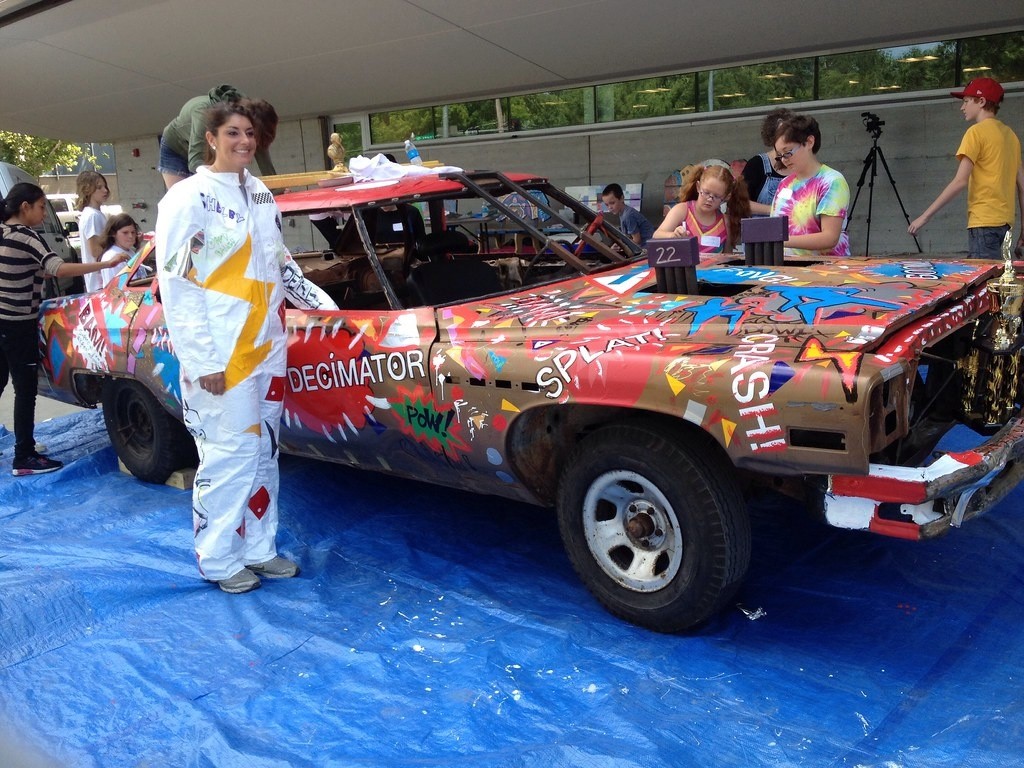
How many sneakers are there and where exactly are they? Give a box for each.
[210,567,262,594]
[244,553,300,579]
[12,452,62,476]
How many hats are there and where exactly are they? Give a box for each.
[951,77,1004,104]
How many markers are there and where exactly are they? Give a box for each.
[683,222,686,231]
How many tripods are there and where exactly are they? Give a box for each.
[843,138,922,257]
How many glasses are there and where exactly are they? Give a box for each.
[699,186,728,203]
[774,144,801,162]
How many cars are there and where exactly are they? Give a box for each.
[36,162,1023,637]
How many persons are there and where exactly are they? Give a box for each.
[907,78,1024,261]
[155,103,340,594]
[0,182,132,477]
[340,202,425,254]
[652,164,751,256]
[75,171,149,294]
[602,183,653,254]
[157,85,292,196]
[738,108,853,258]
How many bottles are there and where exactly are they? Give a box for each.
[404,139,423,167]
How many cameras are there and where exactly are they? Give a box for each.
[861,111,885,138]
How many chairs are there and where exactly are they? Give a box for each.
[317,278,392,311]
[404,229,505,307]
